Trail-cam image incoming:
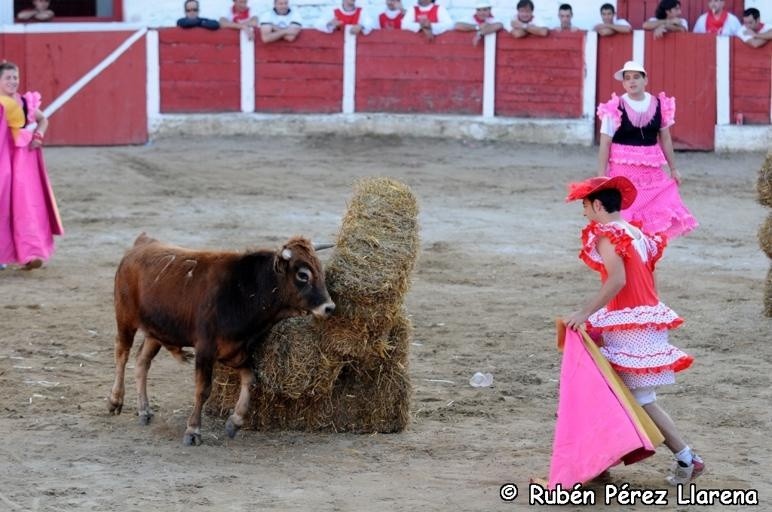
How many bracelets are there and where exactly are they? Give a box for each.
[33,131,44,144]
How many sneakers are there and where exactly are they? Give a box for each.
[667,454,704,488]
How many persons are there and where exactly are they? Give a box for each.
[563,174,706,485]
[258,0,304,43]
[736,7,772,48]
[593,3,632,36]
[453,2,503,48]
[17,0,55,21]
[502,0,550,39]
[643,0,689,40]
[693,0,740,37]
[177,0,219,30]
[596,60,702,240]
[219,0,260,40]
[554,4,579,32]
[313,0,455,43]
[0,59,66,271]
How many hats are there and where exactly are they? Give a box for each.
[612,61,647,81]
[564,175,637,212]
[474,0,491,9]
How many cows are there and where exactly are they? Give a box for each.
[105,230,337,446]
